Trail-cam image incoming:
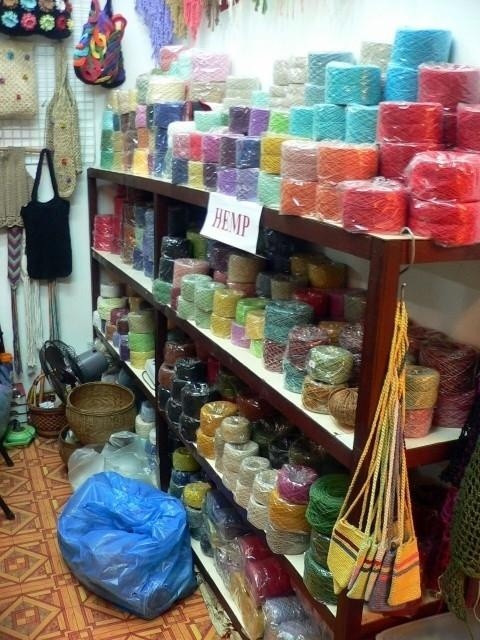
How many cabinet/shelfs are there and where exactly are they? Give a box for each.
[155,177,479,640]
[86,168,160,491]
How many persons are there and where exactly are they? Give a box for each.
[0,327,14,446]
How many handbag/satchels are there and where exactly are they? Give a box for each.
[1,1,72,41]
[328,520,423,611]
[45,83,82,199]
[20,199,71,281]
[74,0,126,88]
[0,35,38,121]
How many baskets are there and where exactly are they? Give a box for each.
[27,374,67,438]
[66,381,135,444]
[58,424,83,469]
